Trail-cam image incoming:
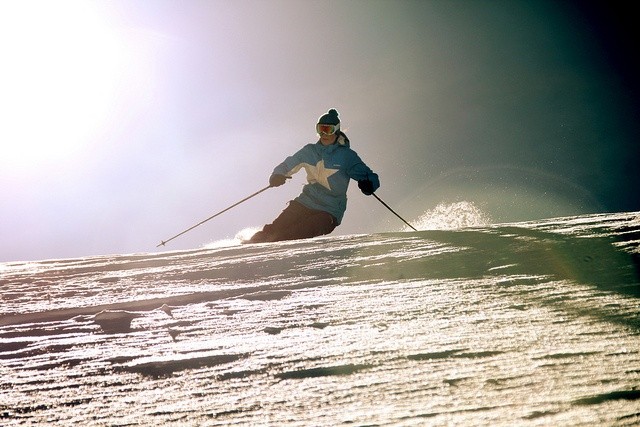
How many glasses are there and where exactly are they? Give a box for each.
[316,123,340,135]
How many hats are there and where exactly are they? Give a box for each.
[319,109,340,125]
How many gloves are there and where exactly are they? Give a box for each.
[359,179,375,196]
[270,175,286,187]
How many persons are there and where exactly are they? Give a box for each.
[250,109,380,243]
